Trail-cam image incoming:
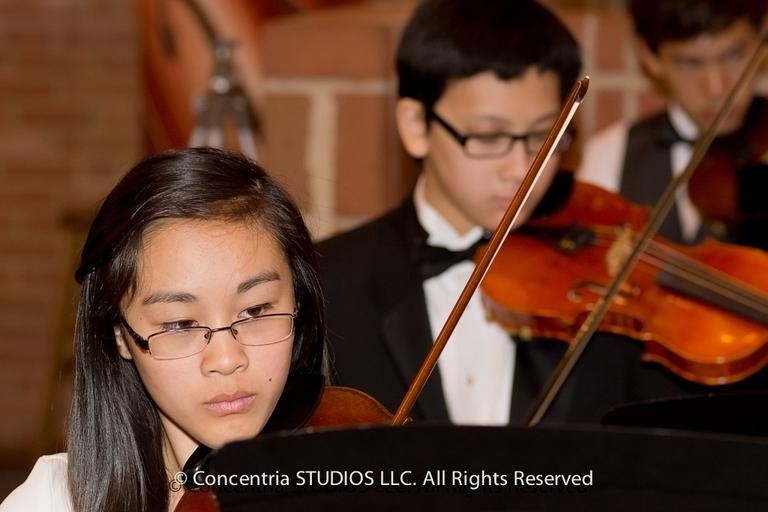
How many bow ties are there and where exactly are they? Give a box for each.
[420,237,485,280]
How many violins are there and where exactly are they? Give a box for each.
[473,176,768,386]
[173,386,422,512]
[683,110,766,225]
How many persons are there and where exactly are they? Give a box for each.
[574,0,768,251]
[0,147,327,512]
[311,0,695,429]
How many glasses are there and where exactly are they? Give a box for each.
[117,302,302,359]
[425,110,573,158]
